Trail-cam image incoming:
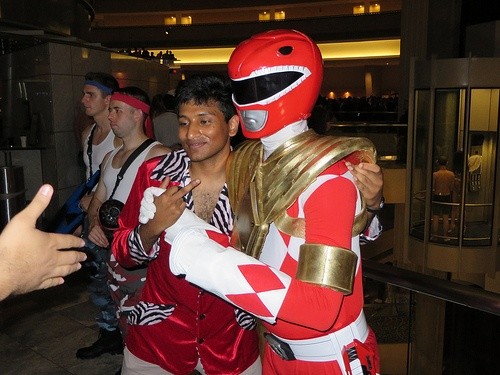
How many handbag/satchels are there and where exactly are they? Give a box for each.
[99,139,154,230]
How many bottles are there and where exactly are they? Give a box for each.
[156,51,163,65]
[141,49,155,62]
[119,48,131,56]
[133,49,141,58]
[163,50,173,68]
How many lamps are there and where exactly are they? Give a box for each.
[164,16,193,26]
[257,7,286,22]
[353,2,381,16]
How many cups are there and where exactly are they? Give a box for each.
[21,136,26,148]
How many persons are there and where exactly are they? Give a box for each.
[428,155,456,242]
[76,84,172,362]
[0,183,87,300]
[70,73,121,237]
[138,27,384,375]
[111,76,384,375]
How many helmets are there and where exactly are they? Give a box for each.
[227,28,323,139]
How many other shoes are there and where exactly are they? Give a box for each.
[77,328,124,359]
